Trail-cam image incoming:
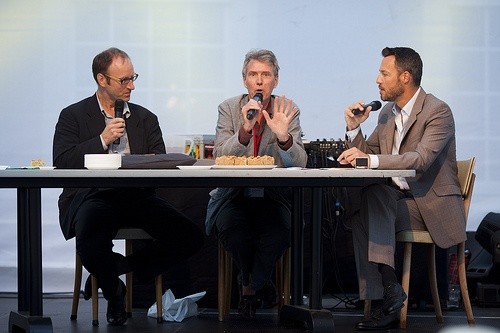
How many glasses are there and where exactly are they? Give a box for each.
[105,72,139,85]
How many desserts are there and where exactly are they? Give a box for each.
[215,155,275,165]
[31,159,45,166]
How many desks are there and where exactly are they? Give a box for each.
[0,166,416,333]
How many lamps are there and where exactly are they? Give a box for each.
[475,212,500,307]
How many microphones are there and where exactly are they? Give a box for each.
[246,92,263,121]
[113,98,125,144]
[351,101,383,116]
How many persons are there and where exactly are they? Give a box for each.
[52,48,194,326]
[206,47,307,318]
[337,47,468,315]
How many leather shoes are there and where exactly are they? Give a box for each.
[105,288,129,325]
[356,312,399,330]
[259,278,281,309]
[239,293,258,319]
[381,283,407,314]
[84,270,99,301]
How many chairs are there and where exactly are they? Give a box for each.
[217,232,293,322]
[70,228,162,328]
[364,157,477,329]
